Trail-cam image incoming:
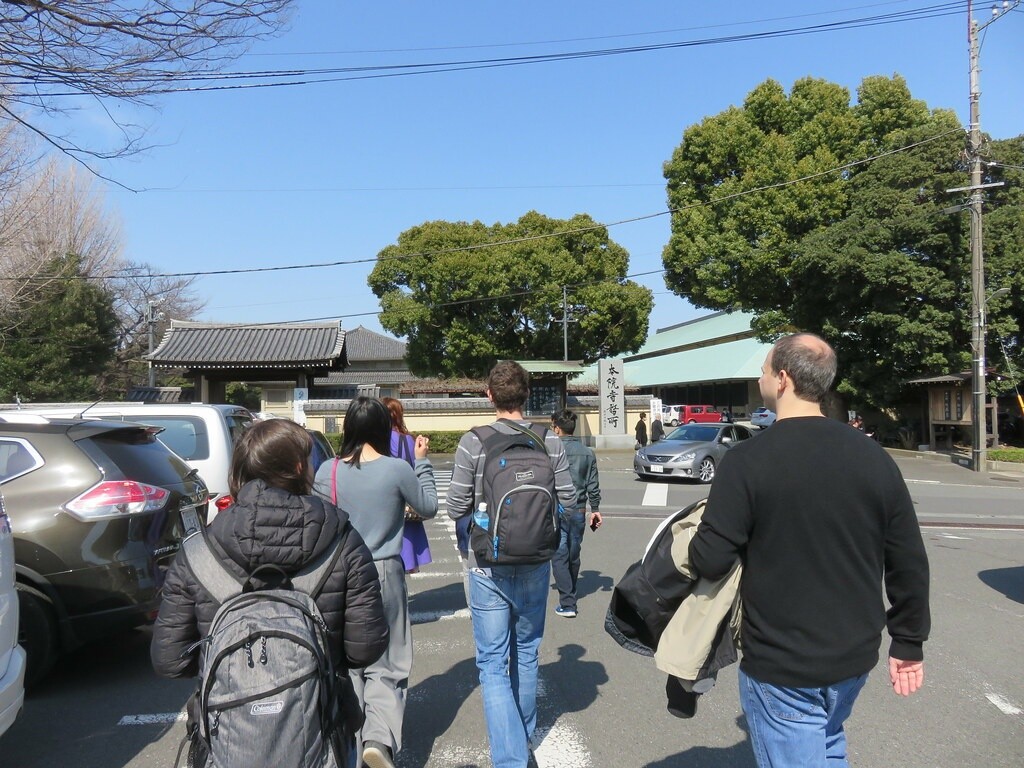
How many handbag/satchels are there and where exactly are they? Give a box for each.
[397,432,435,522]
[635,438,643,450]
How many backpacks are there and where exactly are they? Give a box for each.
[179,517,354,767]
[470,423,561,565]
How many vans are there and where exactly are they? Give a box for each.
[2,398,255,527]
[679,405,722,426]
[663,405,679,427]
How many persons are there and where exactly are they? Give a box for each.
[721,407,734,423]
[635,411,649,448]
[541,409,602,619]
[309,395,439,768]
[446,358,579,768]
[149,417,392,768]
[651,413,665,443]
[721,413,731,424]
[376,398,434,575]
[687,332,930,767]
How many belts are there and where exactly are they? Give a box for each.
[556,508,586,513]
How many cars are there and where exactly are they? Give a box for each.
[0,497,29,735]
[750,406,778,430]
[1,412,215,656]
[633,422,758,485]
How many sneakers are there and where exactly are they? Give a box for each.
[553,605,576,617]
[363,740,396,768]
